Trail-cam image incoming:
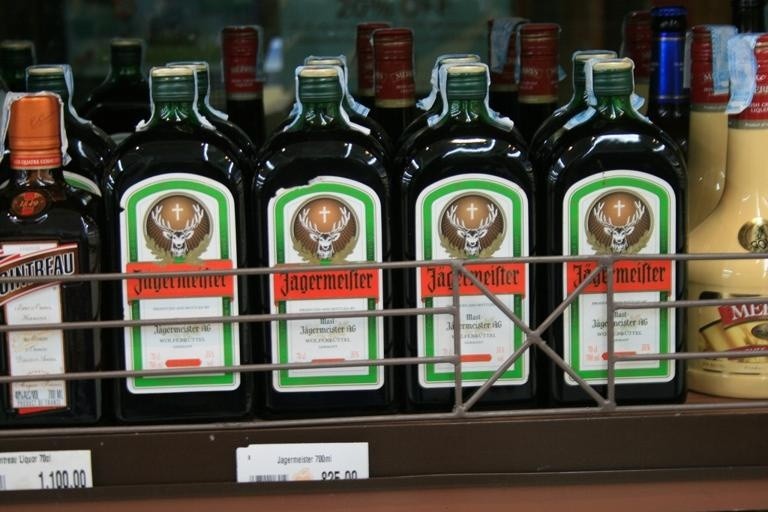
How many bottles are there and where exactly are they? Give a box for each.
[1,6,768,433]
